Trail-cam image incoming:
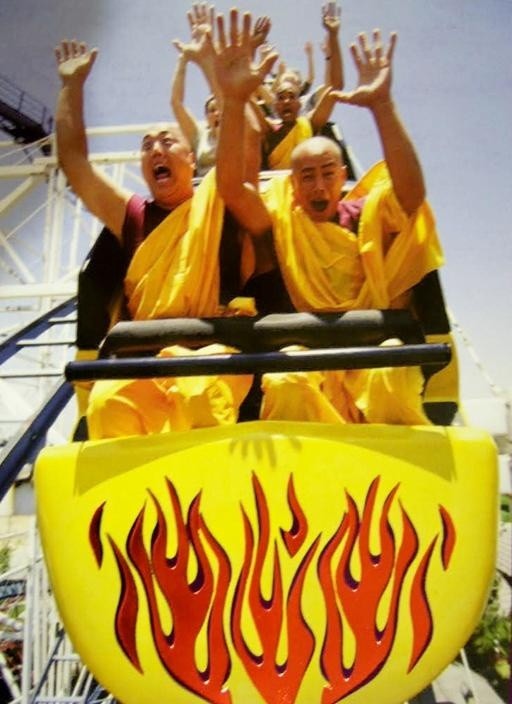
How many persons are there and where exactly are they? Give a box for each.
[51,0,445,443]
